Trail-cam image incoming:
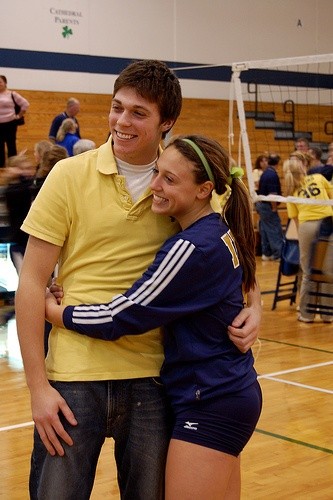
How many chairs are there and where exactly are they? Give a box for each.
[270,215,333,317]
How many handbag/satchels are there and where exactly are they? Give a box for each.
[12,92,25,125]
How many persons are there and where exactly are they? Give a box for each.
[56,118,80,157]
[253,137,333,324]
[15,60,262,500]
[0,141,70,359]
[49,97,80,138]
[44,133,263,500]
[72,139,97,158]
[0,75,30,168]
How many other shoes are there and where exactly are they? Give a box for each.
[273,257,282,262]
[296,305,300,311]
[298,315,313,323]
[323,318,332,322]
[262,254,273,261]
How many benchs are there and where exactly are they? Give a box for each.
[0,89,333,232]
